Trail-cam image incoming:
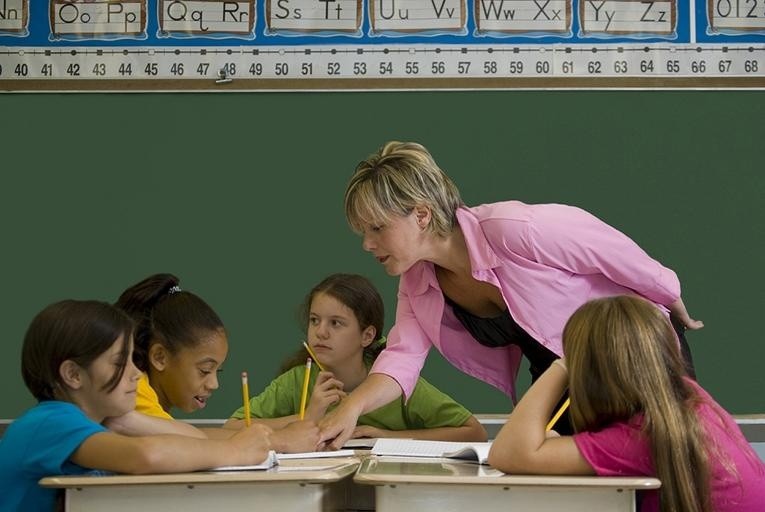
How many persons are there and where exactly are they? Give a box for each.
[103,273,324,455]
[225,273,489,443]
[487,295,765,511]
[318,140,704,464]
[1,299,274,511]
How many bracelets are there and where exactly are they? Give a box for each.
[552,357,571,387]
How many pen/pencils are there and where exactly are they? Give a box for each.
[299,358,312,421]
[300,339,325,372]
[545,397,571,431]
[241,371,251,427]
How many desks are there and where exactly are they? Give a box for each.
[38,448,362,512]
[353,455,666,512]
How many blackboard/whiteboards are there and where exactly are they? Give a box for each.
[0,77,765,442]
